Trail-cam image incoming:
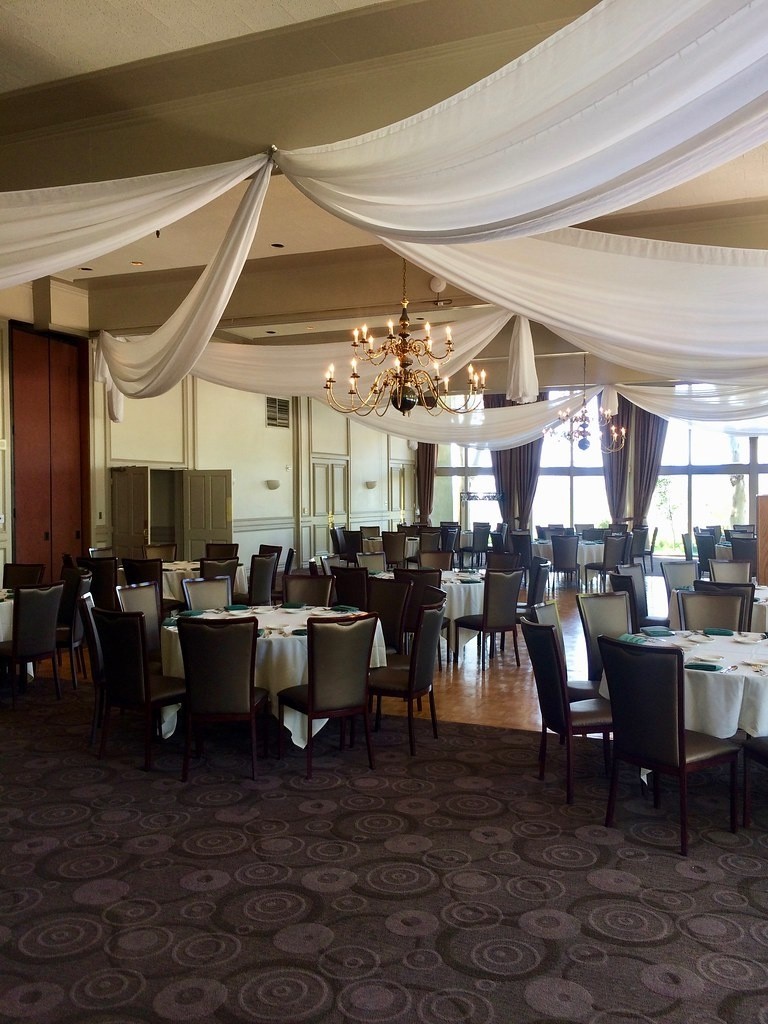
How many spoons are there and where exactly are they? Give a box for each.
[721,665,738,673]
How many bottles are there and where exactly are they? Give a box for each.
[720,534,726,543]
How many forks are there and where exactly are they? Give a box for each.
[752,666,768,676]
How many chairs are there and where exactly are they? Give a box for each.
[0,522,768,856]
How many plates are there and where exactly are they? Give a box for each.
[733,637,760,643]
[191,562,200,564]
[254,607,274,614]
[286,609,306,614]
[174,562,184,564]
[694,655,725,661]
[266,624,290,629]
[688,635,715,643]
[229,611,251,615]
[743,659,768,665]
[311,610,335,616]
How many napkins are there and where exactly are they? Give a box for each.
[640,626,675,636]
[330,605,358,612]
[617,633,646,645]
[684,662,723,671]
[224,605,250,611]
[703,628,733,636]
[461,579,481,584]
[280,603,307,608]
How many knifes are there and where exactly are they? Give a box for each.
[634,634,666,642]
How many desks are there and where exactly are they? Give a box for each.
[162,606,388,749]
[117,562,249,602]
[599,631,768,739]
[378,571,485,653]
[532,540,606,584]
[363,537,419,569]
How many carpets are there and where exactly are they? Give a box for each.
[0,676,768,1024]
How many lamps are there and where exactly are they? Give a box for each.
[266,480,280,490]
[541,354,626,455]
[367,481,377,489]
[323,258,486,417]
